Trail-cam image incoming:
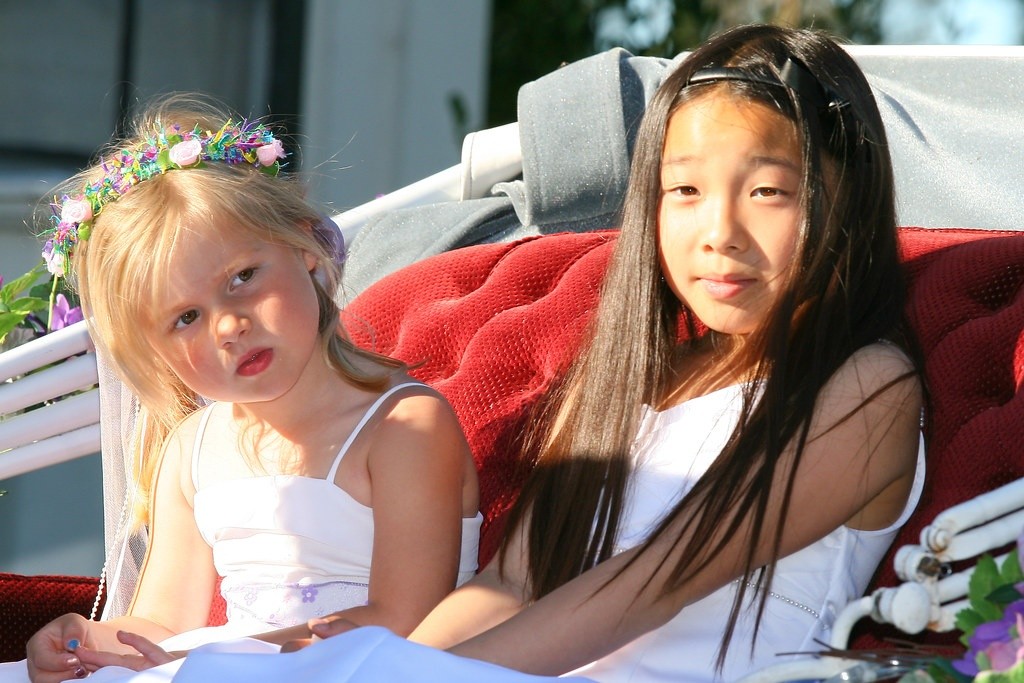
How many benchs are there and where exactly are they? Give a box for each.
[0,226,1024,663]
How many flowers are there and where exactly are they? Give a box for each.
[915,534,1024,683]
[1,257,100,421]
[37,115,283,276]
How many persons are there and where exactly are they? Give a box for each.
[0,92,485,681]
[169,21,930,681]
